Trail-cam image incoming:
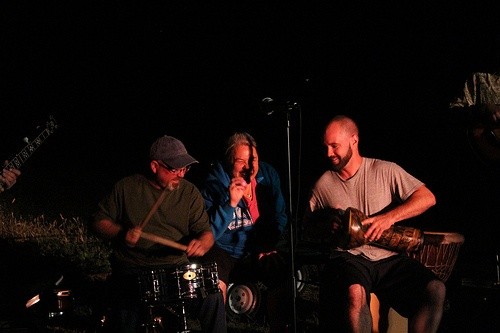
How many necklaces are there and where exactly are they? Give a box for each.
[244,185,251,198]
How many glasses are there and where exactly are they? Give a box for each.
[157,163,191,174]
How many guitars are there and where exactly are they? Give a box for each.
[0,115,59,188]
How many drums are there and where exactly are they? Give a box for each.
[171,262,220,300]
[40,286,108,328]
[137,266,165,304]
[335,205,465,282]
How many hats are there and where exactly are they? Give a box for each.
[149,135,199,170]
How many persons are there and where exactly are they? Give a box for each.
[303,115,446,333]
[200,130,294,333]
[93,135,227,333]
[447,73,500,288]
[0,159,21,192]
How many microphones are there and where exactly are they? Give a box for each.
[258,97,298,116]
